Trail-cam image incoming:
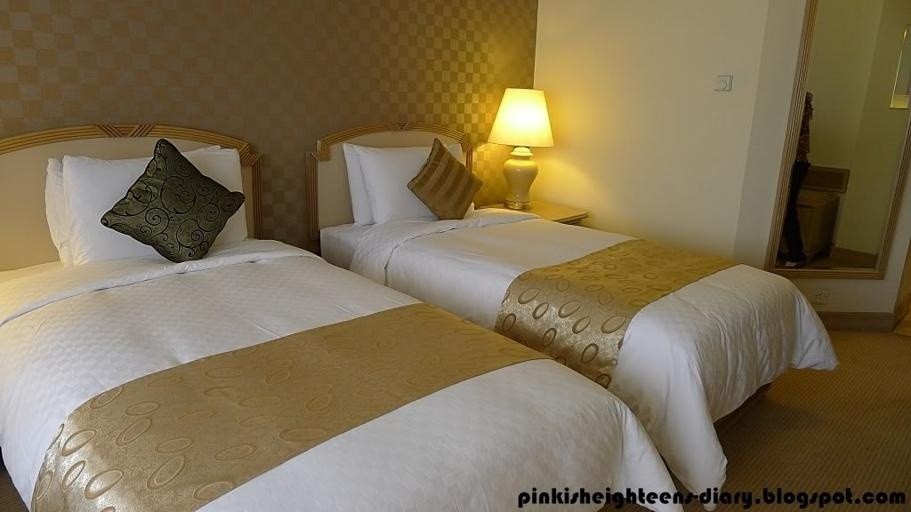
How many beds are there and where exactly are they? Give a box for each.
[0,122,680,512]
[305,120,841,511]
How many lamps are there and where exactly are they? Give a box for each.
[486,85,553,211]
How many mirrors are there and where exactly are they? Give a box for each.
[765,0,909,280]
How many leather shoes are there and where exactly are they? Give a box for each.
[782,258,806,269]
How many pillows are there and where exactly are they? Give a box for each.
[44,143,223,265]
[62,144,245,265]
[354,141,470,223]
[410,135,485,220]
[101,136,248,261]
[343,141,373,226]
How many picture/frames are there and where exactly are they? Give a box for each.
[888,22,910,110]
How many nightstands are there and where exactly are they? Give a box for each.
[479,201,588,225]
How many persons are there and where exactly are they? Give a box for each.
[775,90,813,267]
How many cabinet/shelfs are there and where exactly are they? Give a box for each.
[791,187,842,261]
